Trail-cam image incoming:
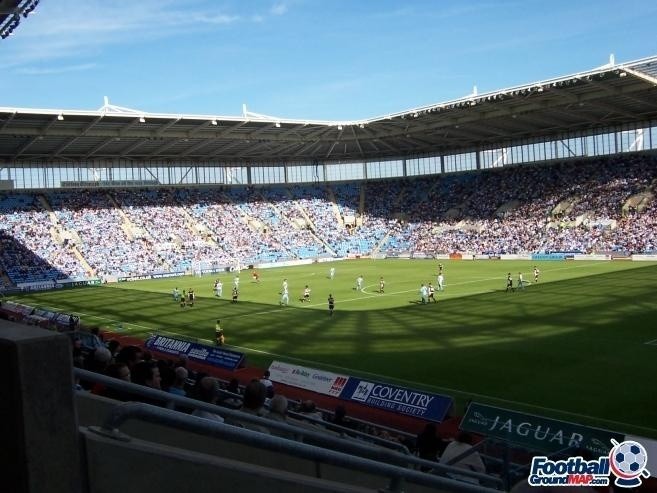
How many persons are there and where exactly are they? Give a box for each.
[0,150,657,493]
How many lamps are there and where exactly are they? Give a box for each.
[399,68,620,122]
[0,0,41,41]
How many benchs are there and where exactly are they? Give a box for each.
[24,326,654,492]
[0,156,656,309]
